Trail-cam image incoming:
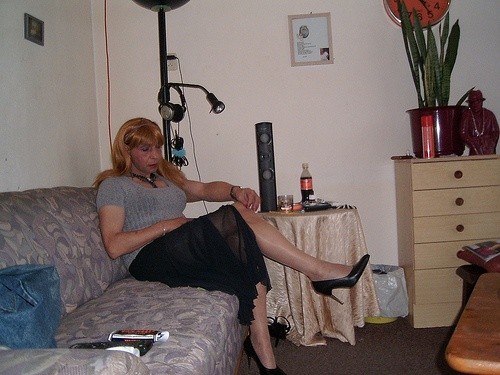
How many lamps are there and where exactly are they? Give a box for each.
[134,0,225,164]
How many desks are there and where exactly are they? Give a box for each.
[444,271,500,375]
[254,208,383,347]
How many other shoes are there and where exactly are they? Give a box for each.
[266,316,291,348]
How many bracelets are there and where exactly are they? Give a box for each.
[230,185,240,202]
[160,221,166,236]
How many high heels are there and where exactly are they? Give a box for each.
[312,253,370,306]
[243,335,286,375]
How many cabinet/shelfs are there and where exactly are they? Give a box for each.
[394,154,500,329]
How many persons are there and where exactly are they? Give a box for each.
[91,118,370,375]
[460,90,499,156]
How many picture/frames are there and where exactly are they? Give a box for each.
[24,13,44,46]
[287,12,334,67]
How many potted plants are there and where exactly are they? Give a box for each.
[396,0,469,160]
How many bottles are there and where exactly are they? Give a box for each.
[421,114,435,158]
[300,163,314,203]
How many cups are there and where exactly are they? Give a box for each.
[278,195,293,213]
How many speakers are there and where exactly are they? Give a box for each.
[255,121,277,212]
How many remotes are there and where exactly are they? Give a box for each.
[68,340,153,356]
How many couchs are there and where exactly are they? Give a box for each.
[0,187,249,375]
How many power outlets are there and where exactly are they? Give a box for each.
[167,53,179,71]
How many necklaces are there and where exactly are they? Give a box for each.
[466,106,485,137]
[128,171,158,189]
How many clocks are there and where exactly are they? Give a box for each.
[383,0,452,31]
